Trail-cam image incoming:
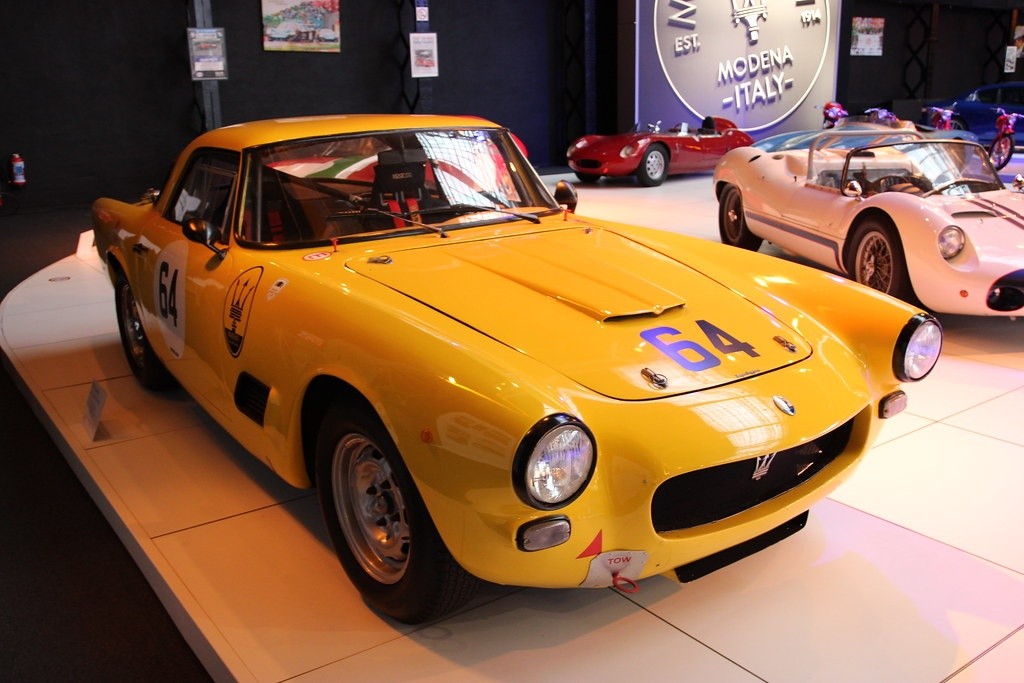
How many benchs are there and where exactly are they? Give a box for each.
[816,160,922,198]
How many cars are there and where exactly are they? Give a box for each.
[922,81,1024,148]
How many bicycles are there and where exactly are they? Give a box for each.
[921,101,959,132]
[986,107,1024,171]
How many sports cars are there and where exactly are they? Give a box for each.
[91,114,946,626]
[573,114,757,186]
[712,114,1024,323]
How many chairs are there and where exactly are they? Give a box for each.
[373,149,453,229]
[213,176,312,245]
[698,116,715,134]
